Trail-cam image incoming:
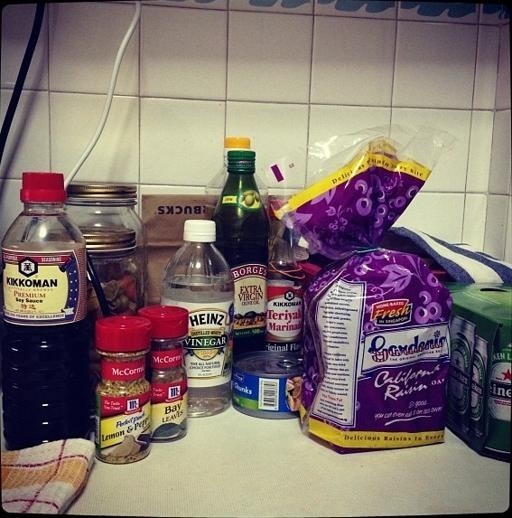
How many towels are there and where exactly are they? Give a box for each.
[0,436,97,517]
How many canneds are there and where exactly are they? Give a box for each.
[230,351,305,420]
[470,334,488,438]
[446,313,476,435]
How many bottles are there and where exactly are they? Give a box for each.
[2,163,92,447]
[168,219,232,414]
[94,306,196,470]
[218,139,313,372]
